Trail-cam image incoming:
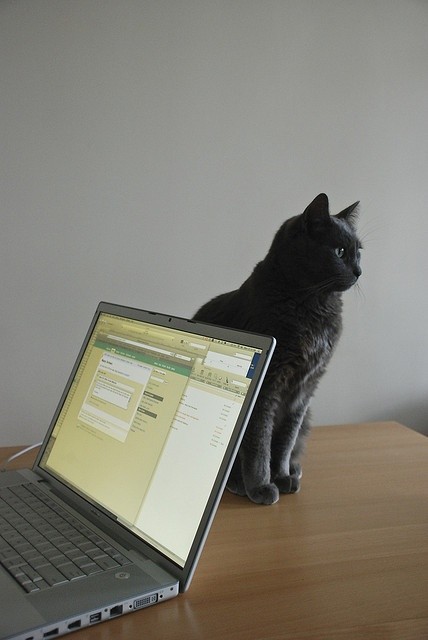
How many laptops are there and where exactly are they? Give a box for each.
[0,300,277,639]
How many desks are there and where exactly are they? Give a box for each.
[0,419,427,636]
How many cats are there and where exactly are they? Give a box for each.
[190,193,364,505]
